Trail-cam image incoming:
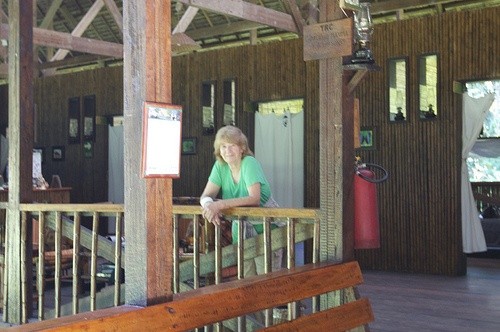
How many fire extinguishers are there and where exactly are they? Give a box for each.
[351,152,389,250]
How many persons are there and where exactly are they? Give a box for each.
[199,126,284,323]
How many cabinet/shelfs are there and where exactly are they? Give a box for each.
[1,187,72,249]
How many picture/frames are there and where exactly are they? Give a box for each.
[142,101,183,178]
